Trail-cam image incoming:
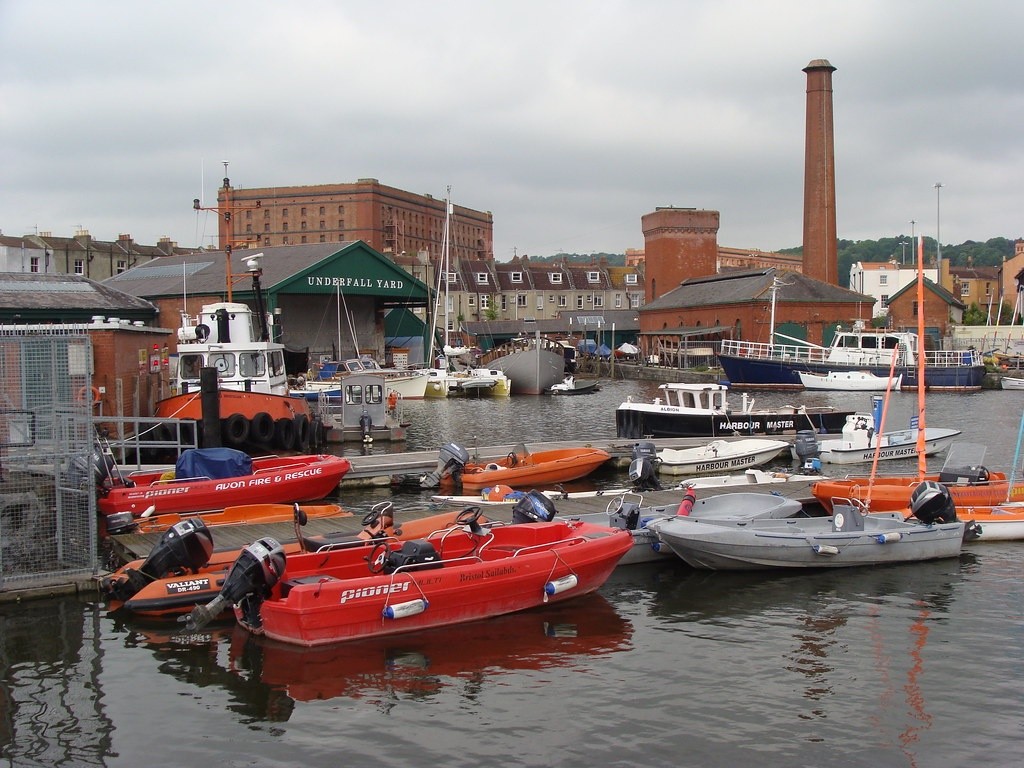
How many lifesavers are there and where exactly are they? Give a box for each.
[157,422,180,451]
[275,417,297,451]
[178,420,203,449]
[309,420,322,451]
[76,385,101,409]
[294,414,311,450]
[253,411,275,442]
[224,413,250,445]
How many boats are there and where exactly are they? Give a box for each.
[998,376,1023,389]
[390,440,612,494]
[864,507,1024,541]
[288,272,430,400]
[544,379,597,397]
[41,449,350,518]
[577,339,642,360]
[813,425,960,465]
[147,160,328,458]
[317,371,409,446]
[797,369,903,391]
[814,235,1024,510]
[716,268,987,391]
[644,512,964,566]
[617,382,856,440]
[511,485,805,571]
[98,498,635,646]
[620,432,790,476]
[479,331,566,396]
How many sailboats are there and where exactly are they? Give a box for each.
[410,184,513,399]
